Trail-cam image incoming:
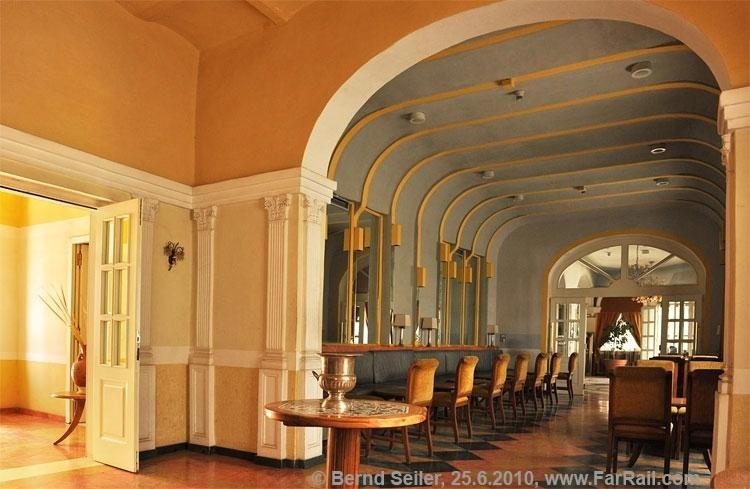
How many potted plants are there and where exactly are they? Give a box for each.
[594,317,636,375]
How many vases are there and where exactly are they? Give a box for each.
[71,343,87,394]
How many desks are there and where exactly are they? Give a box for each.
[48,391,90,447]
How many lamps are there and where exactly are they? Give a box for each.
[512,193,525,203]
[654,176,671,187]
[651,141,668,156]
[408,110,427,125]
[480,169,495,180]
[630,260,662,307]
[629,59,652,80]
[485,324,501,348]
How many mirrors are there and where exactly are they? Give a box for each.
[322,193,487,347]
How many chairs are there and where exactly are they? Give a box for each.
[263,347,724,489]
